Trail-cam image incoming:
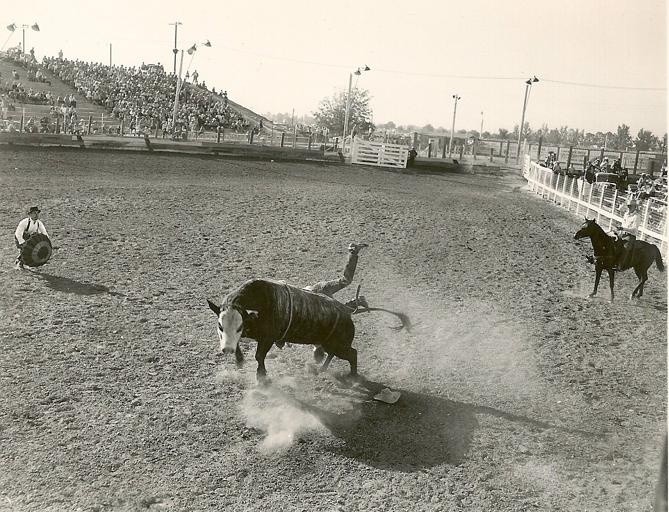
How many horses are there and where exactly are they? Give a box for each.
[573,216,665,303]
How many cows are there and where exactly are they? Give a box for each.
[206,277,413,381]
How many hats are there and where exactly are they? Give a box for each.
[27,206,41,215]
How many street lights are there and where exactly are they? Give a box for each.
[6,22,39,54]
[167,21,185,69]
[342,63,372,153]
[479,112,485,138]
[448,95,463,156]
[516,75,539,166]
[172,40,212,135]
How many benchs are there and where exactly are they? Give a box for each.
[1,59,134,135]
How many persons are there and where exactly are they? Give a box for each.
[294,121,375,145]
[14,206,49,270]
[301,242,373,364]
[612,200,643,274]
[537,146,667,240]
[408,147,419,167]
[0,41,272,146]
